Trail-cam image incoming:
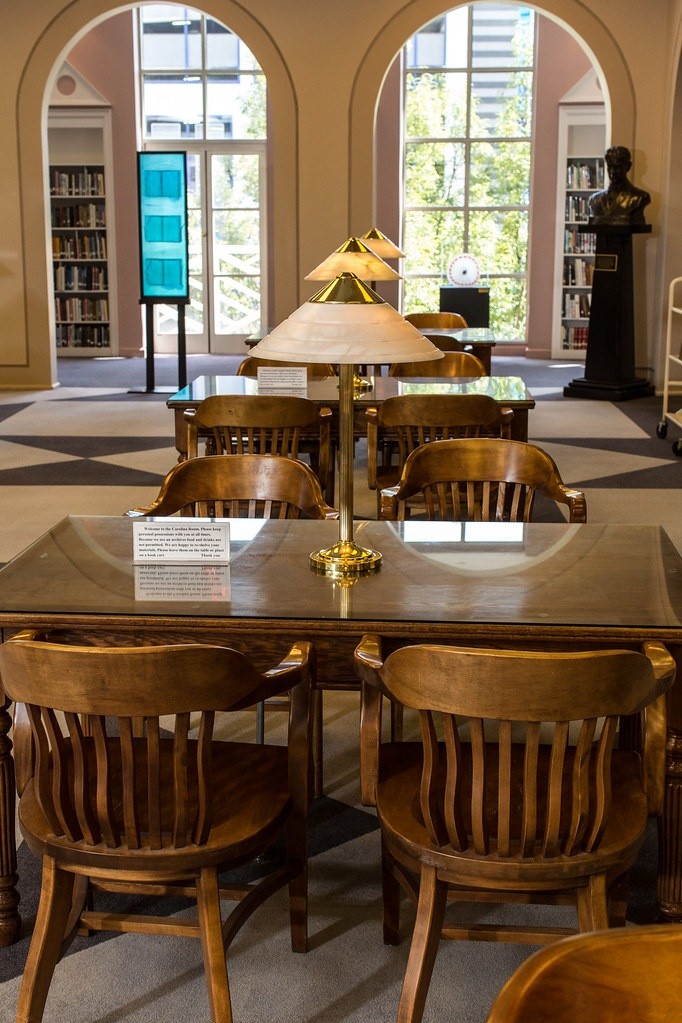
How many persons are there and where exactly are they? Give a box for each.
[589,146,650,214]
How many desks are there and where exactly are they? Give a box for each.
[0,512,682,951]
[244,327,497,377]
[166,376,536,522]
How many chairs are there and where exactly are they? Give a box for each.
[0,313,682,1023]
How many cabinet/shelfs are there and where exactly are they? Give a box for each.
[551,103,607,359]
[655,276,682,460]
[46,108,120,358]
[439,285,491,376]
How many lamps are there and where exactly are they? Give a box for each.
[358,228,407,292]
[303,235,404,392]
[247,273,444,575]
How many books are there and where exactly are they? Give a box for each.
[562,326,588,349]
[52,203,105,227]
[55,296,109,321]
[56,324,110,347]
[565,196,593,221]
[563,293,591,317]
[50,167,105,196]
[563,259,594,285]
[53,230,107,259]
[565,230,596,254]
[54,263,107,290]
[567,159,604,188]
